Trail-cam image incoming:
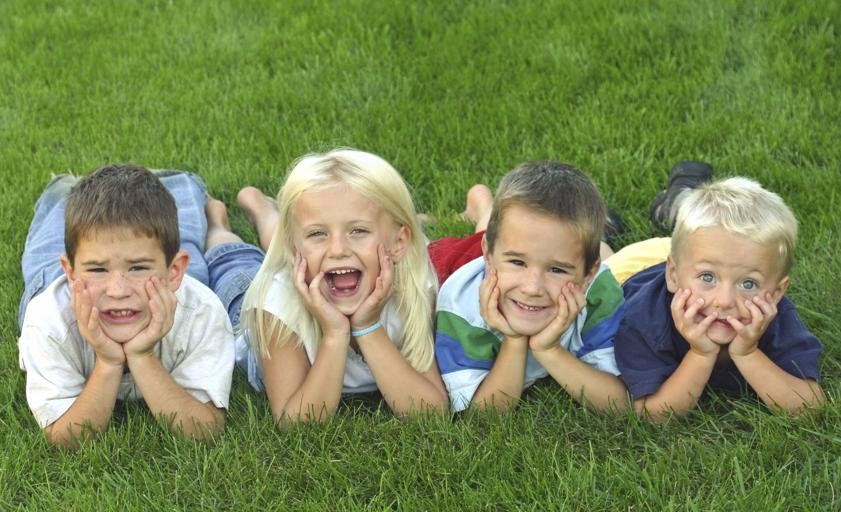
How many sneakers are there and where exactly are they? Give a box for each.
[601,204,624,240]
[648,161,713,234]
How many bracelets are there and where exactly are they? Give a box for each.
[347,320,383,339]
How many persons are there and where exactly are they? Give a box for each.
[411,159,632,431]
[205,147,450,432]
[17,163,235,451]
[591,158,826,429]
